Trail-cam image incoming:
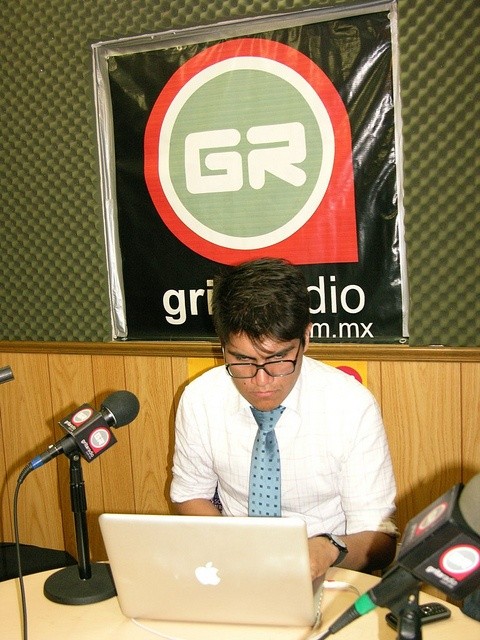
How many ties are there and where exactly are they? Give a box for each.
[248,406,286,517]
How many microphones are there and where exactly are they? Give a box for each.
[329,473,480,640]
[16,390,140,477]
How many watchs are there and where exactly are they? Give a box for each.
[316,533,349,566]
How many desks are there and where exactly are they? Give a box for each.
[0,564,480,640]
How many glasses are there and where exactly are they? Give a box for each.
[221,335,302,378]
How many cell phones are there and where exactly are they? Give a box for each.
[386,602,451,632]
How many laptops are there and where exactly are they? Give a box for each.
[98,513,325,627]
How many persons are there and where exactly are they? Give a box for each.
[169,257,398,584]
